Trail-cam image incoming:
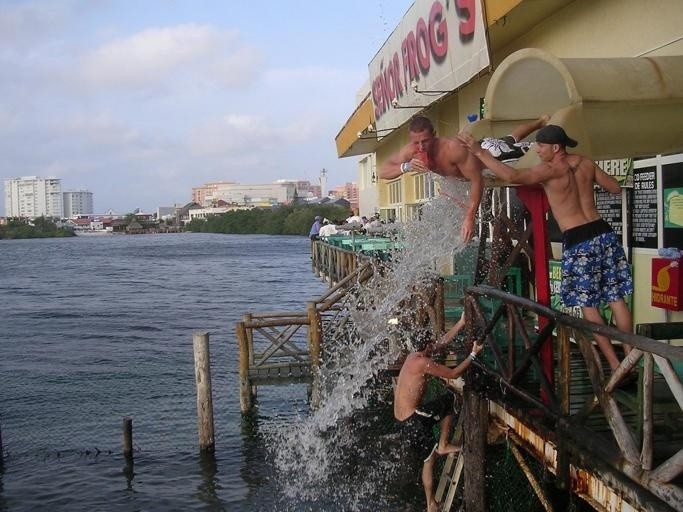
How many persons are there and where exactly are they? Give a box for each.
[379,115,553,243]
[309,213,394,243]
[393,306,483,512]
[457,125,639,388]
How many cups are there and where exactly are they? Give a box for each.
[413,150,430,172]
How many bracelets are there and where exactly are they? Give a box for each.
[468,352,477,361]
[401,162,409,174]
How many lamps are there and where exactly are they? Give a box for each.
[356,130,387,140]
[410,81,454,94]
[367,123,400,134]
[391,97,432,109]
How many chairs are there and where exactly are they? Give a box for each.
[329,230,522,343]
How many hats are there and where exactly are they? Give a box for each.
[536,125,577,147]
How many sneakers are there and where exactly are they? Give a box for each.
[606,370,639,387]
